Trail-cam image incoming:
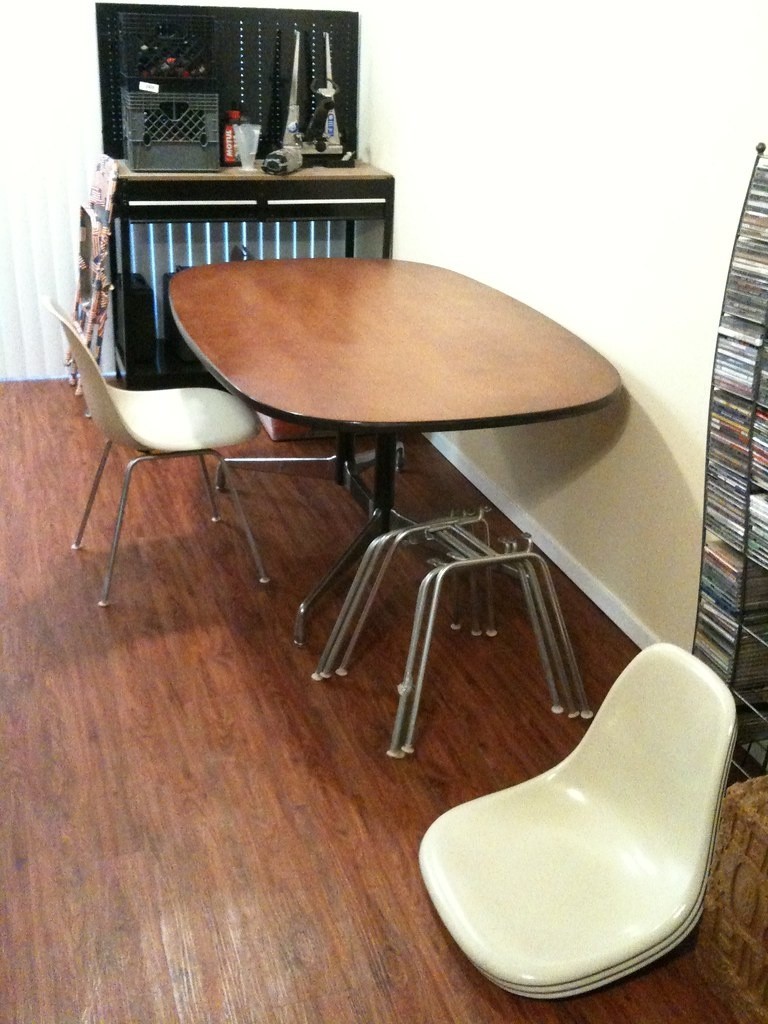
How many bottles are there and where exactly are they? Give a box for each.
[223,110,244,166]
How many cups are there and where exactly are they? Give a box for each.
[232,123,261,171]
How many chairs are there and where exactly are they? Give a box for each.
[312,501,742,999]
[43,293,271,611]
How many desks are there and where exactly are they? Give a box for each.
[111,160,395,389]
[167,255,625,651]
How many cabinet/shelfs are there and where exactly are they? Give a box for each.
[688,142,768,789]
